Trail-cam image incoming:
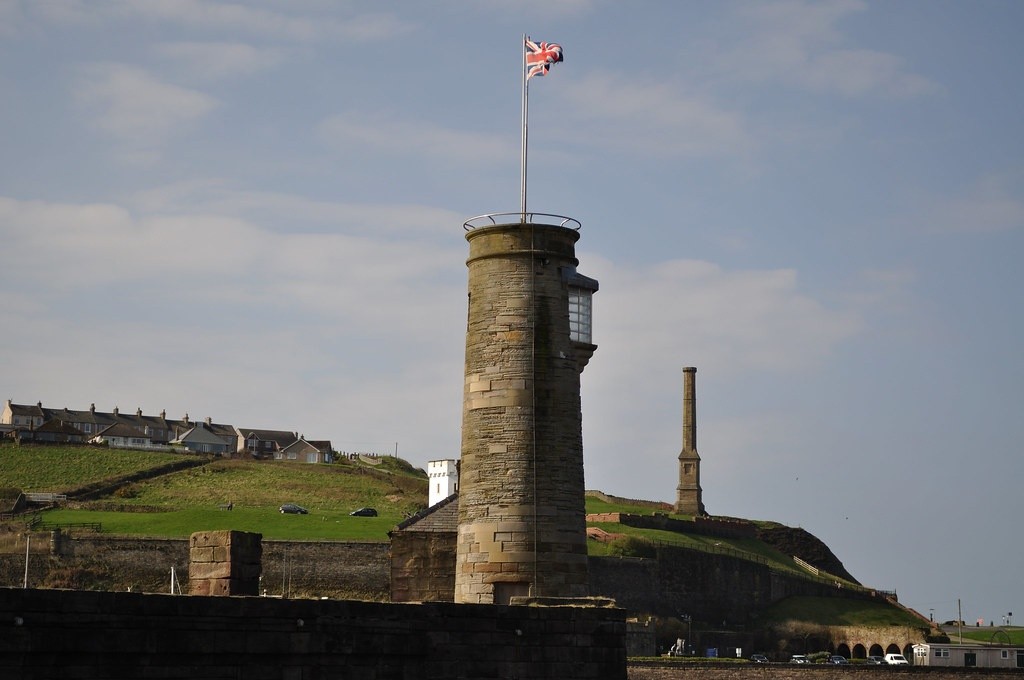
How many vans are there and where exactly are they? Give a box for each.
[885,654,908,665]
[280,503,308,516]
[866,656,888,665]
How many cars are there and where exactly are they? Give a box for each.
[750,654,769,663]
[789,655,811,664]
[831,655,849,664]
[352,508,378,517]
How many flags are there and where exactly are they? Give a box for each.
[525,40,563,81]
